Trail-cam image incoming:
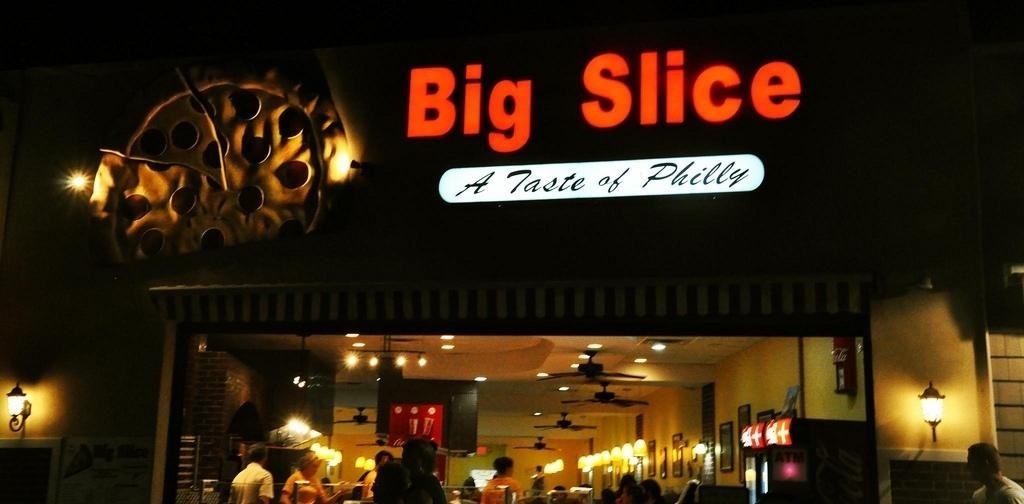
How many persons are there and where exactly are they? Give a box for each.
[967,442,1023,503]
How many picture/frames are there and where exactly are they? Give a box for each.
[646,402,797,484]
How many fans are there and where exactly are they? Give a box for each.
[534,348,647,381]
[513,437,563,453]
[332,406,377,425]
[533,411,597,432]
[559,380,649,409]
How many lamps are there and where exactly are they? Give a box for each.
[6,381,32,432]
[918,379,946,443]
[344,334,429,372]
[578,387,646,471]
